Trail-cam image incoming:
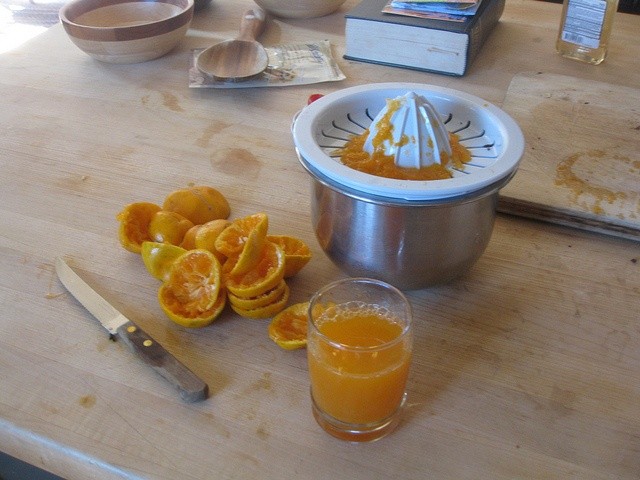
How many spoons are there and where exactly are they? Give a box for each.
[194,9,269,80]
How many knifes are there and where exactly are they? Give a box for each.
[50,257,210,404]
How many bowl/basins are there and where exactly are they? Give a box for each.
[254,0,349,19]
[56,0,196,63]
[293,97,523,292]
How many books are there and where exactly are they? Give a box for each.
[342,1,507,79]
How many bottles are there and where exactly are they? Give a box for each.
[552,1,619,67]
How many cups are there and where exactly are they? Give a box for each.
[306,277,416,444]
